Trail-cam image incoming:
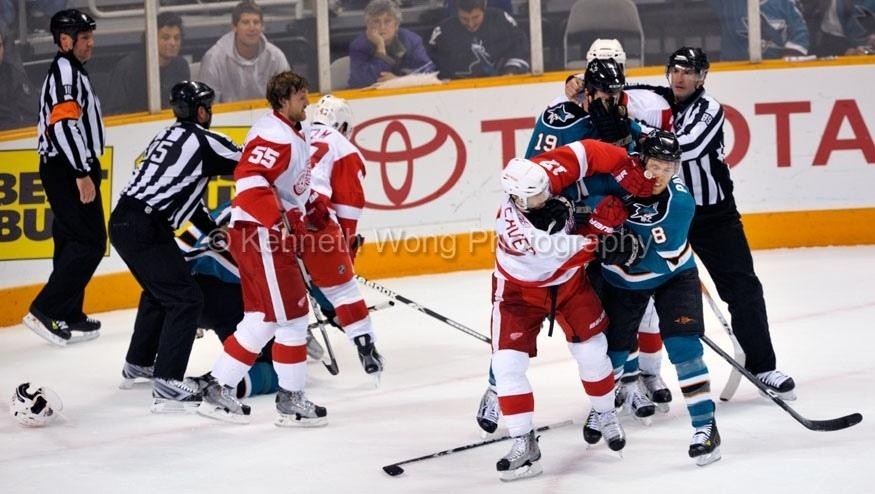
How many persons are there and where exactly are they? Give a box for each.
[175,94,385,398]
[202,69,330,419]
[0,33,40,132]
[22,9,107,343]
[347,0,436,90]
[814,0,875,57]
[107,80,243,402]
[426,0,530,82]
[476,38,796,432]
[109,11,191,117]
[543,130,721,457]
[715,0,811,61]
[196,2,292,103]
[491,139,656,469]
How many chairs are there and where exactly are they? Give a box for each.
[331,57,351,90]
[563,0,644,69]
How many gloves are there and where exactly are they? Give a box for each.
[588,196,627,236]
[346,234,364,262]
[306,190,329,228]
[612,154,656,198]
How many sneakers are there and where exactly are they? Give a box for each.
[122,327,383,419]
[27,306,72,339]
[67,312,100,332]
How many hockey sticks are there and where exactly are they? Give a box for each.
[701,282,746,401]
[702,336,863,431]
[272,185,339,376]
[383,421,573,476]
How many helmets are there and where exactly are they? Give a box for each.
[13,382,58,426]
[666,47,709,88]
[639,130,682,174]
[170,80,214,129]
[501,158,549,212]
[585,38,626,112]
[49,9,96,54]
[312,94,354,142]
